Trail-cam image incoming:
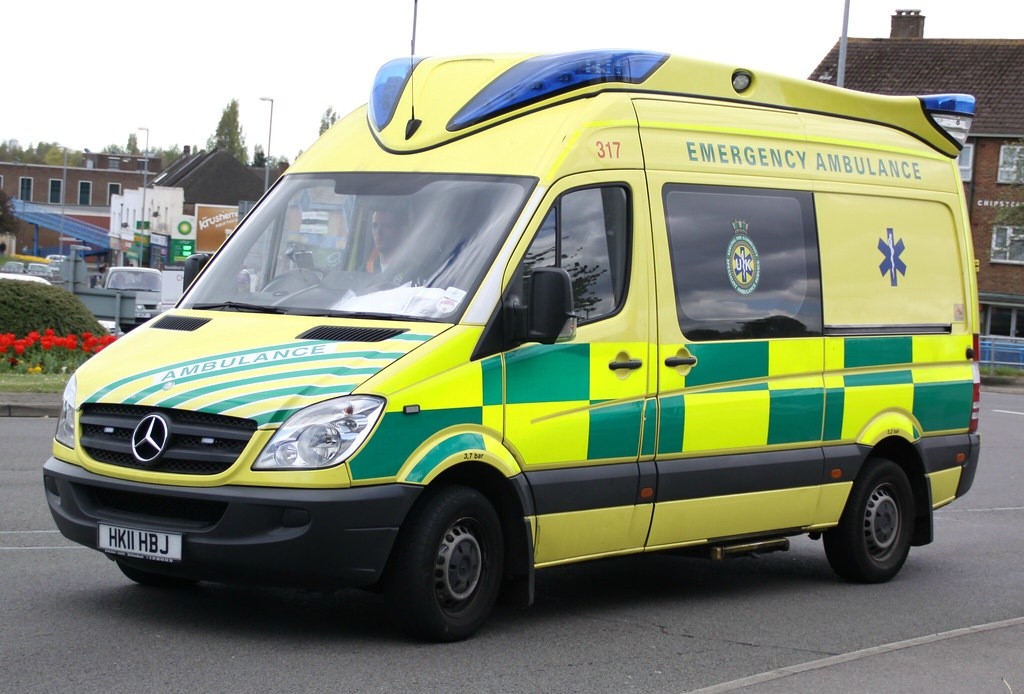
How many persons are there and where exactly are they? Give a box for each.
[356,203,406,275]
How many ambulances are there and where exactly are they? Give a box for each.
[42,46,983,647]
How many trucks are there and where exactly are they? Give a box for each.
[91,265,163,329]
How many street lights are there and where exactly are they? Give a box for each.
[259,97,273,291]
[135,126,149,267]
[52,144,69,262]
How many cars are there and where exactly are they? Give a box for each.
[25,264,53,278]
[97,320,124,337]
[1,262,25,274]
